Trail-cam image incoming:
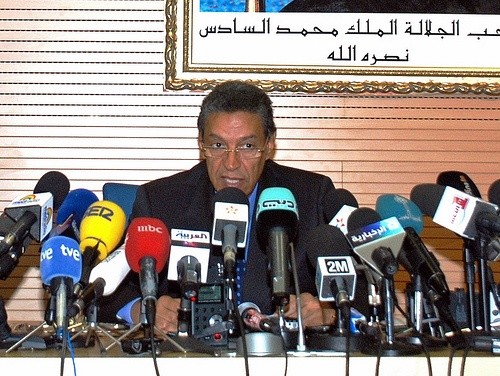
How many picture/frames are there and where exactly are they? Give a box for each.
[164,0,500,95]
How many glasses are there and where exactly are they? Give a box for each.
[200,128,271,159]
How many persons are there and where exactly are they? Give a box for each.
[83,81,396,339]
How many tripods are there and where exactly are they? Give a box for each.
[4,282,187,355]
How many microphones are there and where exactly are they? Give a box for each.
[0,171,500,340]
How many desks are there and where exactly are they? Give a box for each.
[0,324,500,376]
